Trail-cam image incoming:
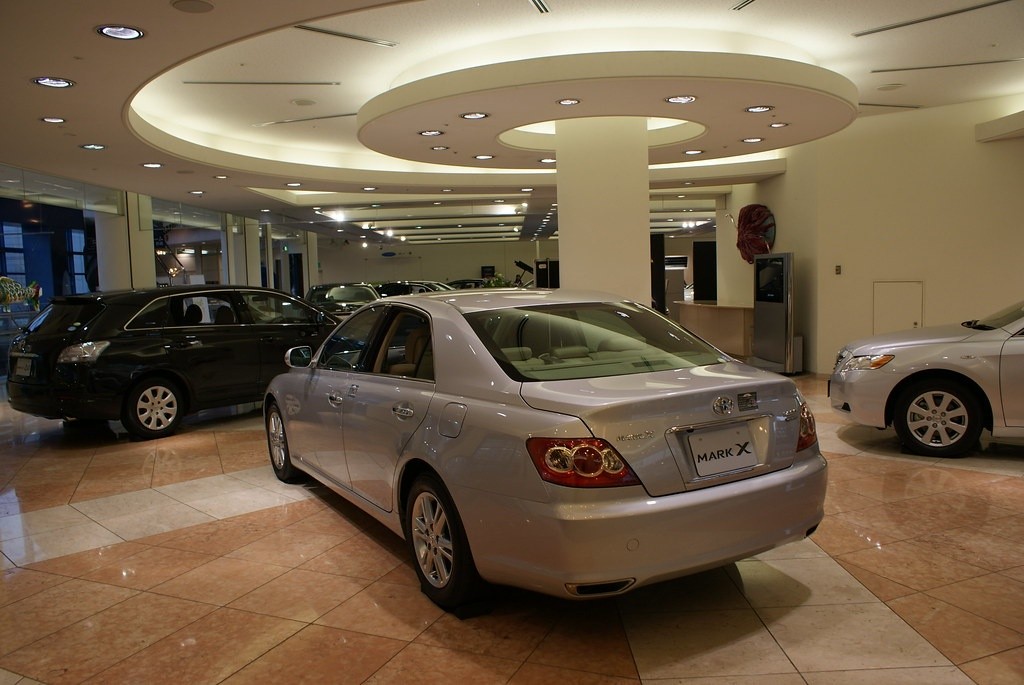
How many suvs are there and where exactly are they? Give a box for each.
[297,283,388,340]
[371,279,456,301]
[446,278,493,290]
[6,281,364,442]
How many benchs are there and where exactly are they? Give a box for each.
[490,334,649,368]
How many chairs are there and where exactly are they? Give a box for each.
[387,326,433,377]
[183,304,202,324]
[215,305,234,324]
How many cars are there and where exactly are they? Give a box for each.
[262,287,828,614]
[827,300,1024,458]
